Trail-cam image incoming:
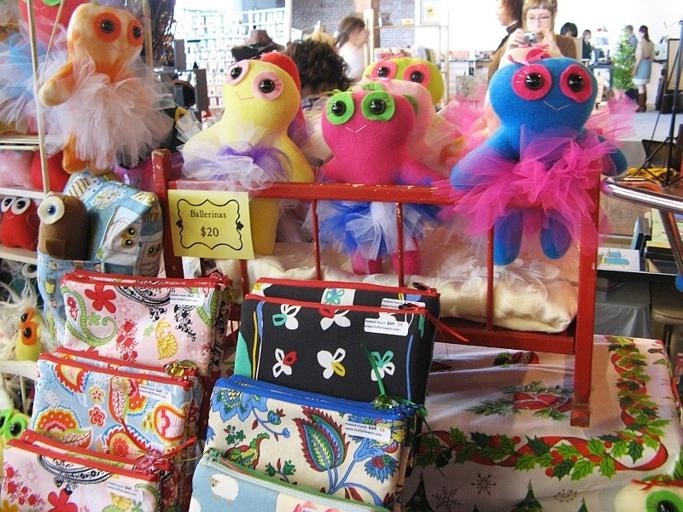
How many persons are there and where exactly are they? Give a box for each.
[339,15,368,83]
[488,1,590,83]
[631,27,654,112]
[624,25,637,60]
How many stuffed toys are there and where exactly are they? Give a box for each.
[610,475,683,512]
[0,408,31,447]
[14,307,46,360]
[185,34,629,275]
[0,0,176,261]
[0,0,628,275]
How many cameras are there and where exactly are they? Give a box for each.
[523,31,544,44]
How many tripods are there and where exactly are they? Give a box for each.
[632,23,683,191]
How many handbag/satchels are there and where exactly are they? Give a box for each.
[188,276,440,512]
[1,270,234,512]
